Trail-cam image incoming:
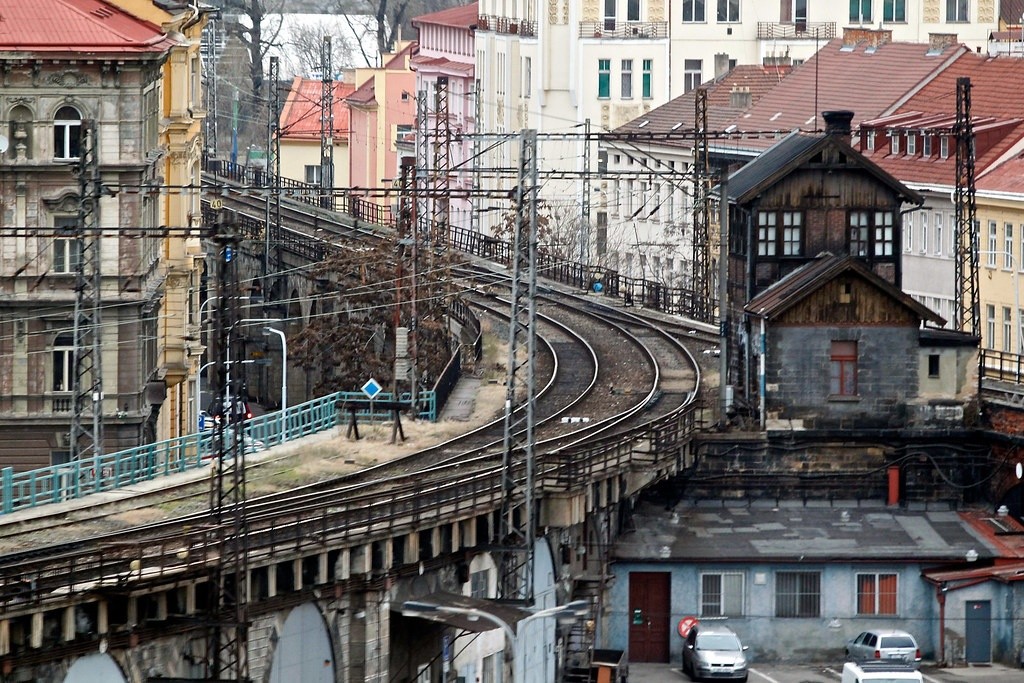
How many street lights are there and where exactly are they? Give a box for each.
[403,599,590,683]
[261,325,287,446]
[195,359,255,437]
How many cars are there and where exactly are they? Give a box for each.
[200,411,214,434]
[682,624,749,683]
[209,394,251,436]
[845,628,924,674]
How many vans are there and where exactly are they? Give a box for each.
[841,660,925,683]
